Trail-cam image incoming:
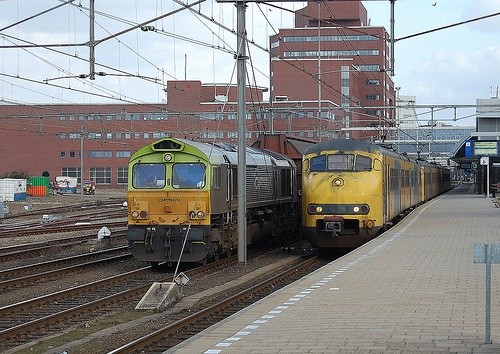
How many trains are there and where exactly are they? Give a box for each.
[126,134,320,272]
[302,140,451,248]
